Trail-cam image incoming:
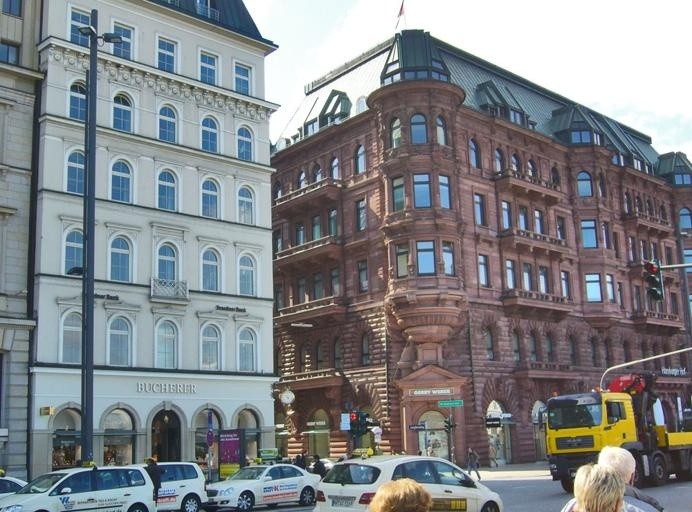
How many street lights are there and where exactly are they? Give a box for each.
[76,6,125,464]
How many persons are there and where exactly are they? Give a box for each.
[429,431,442,457]
[574,464,625,512]
[490,434,501,459]
[560,445,664,512]
[294,449,327,478]
[147,453,165,501]
[391,444,499,482]
[369,477,434,512]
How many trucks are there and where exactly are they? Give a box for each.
[538,373,691,492]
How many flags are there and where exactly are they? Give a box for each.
[397,1,405,16]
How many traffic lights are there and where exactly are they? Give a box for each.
[645,258,665,301]
[444,420,450,432]
[350,411,370,435]
